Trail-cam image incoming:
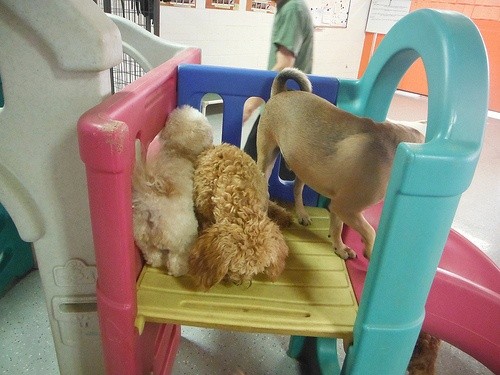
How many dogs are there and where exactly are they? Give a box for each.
[131,104,295,289]
[256,68,425,259]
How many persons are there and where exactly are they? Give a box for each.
[131,0,154,25]
[243,0,314,182]
